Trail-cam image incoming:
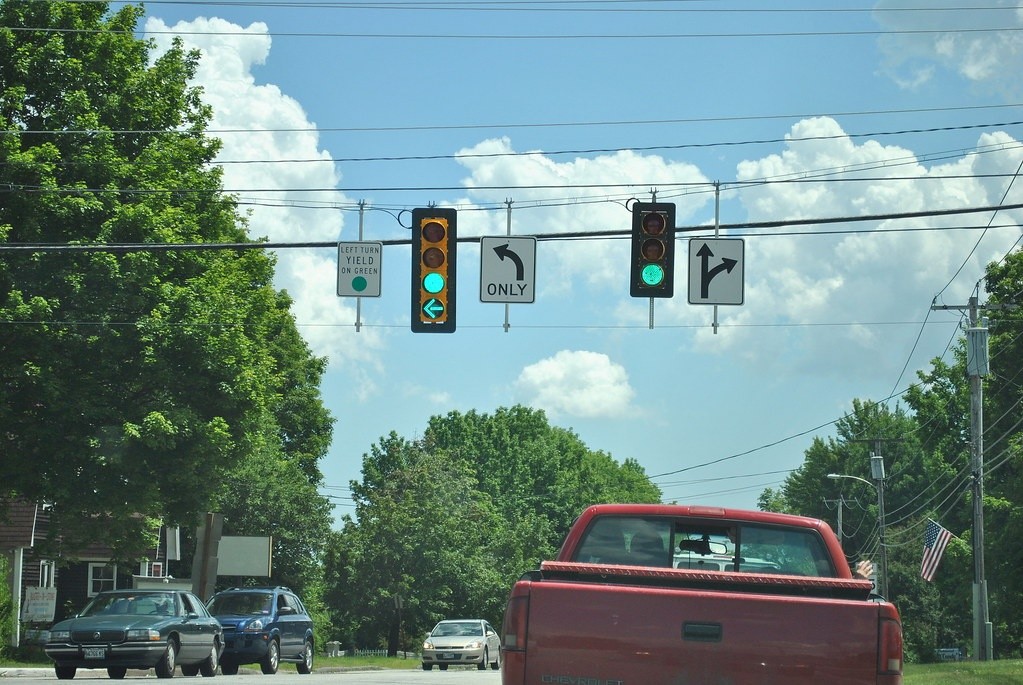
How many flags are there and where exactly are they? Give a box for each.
[920,519,952,582]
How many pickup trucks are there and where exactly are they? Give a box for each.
[499,503,905,684]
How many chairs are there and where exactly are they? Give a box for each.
[597,549,669,568]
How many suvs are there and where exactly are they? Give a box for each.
[201,586,315,675]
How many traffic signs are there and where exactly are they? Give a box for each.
[480,236,536,304]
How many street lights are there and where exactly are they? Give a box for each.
[825,472,890,604]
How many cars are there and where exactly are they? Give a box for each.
[420,618,501,671]
[44,589,225,679]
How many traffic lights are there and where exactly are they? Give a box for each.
[411,208,456,331]
[630,201,675,297]
